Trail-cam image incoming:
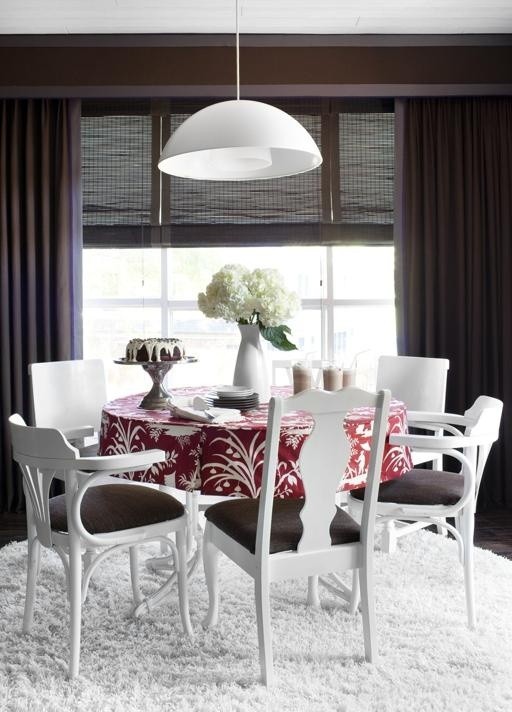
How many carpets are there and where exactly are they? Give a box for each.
[0,519,512,711]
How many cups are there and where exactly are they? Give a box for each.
[292,366,312,395]
[322,363,343,391]
[343,357,357,386]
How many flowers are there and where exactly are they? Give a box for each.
[197,262,300,353]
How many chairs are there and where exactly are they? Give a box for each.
[29,358,109,450]
[9,411,194,679]
[347,354,504,632]
[200,388,393,686]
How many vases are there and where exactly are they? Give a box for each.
[230,323,272,407]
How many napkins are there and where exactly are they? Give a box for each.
[164,396,245,426]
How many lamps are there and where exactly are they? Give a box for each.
[155,0,324,184]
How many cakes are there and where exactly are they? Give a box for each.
[125,337,186,363]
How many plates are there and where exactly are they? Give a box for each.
[205,385,259,412]
